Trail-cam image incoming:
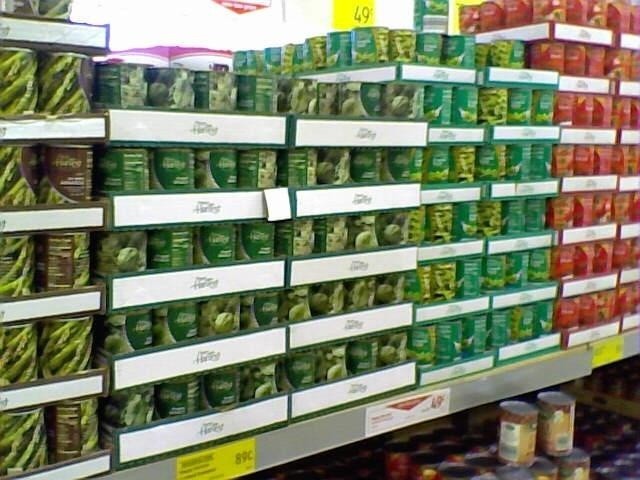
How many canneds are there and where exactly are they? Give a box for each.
[0,0,640,480]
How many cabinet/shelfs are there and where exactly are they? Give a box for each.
[0,0,640,480]
